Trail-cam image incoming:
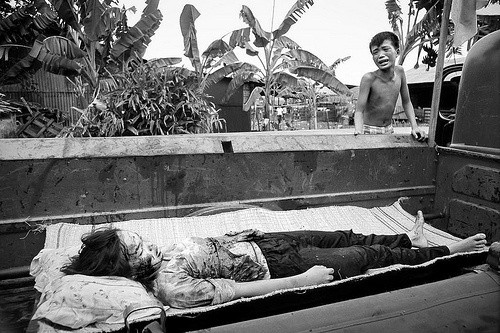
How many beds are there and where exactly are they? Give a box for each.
[20,197,490,333]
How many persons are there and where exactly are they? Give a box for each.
[262,103,290,125]
[354,31,432,144]
[55,210,486,311]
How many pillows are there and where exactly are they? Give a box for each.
[30,248,170,329]
